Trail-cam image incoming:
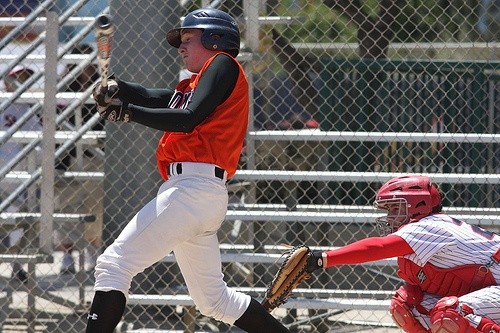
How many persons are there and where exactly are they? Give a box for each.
[263,173,500,333]
[44,44,105,276]
[1,65,47,283]
[86,8,296,333]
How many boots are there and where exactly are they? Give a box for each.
[234,293,292,333]
[85,289,127,332]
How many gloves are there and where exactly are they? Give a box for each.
[93,72,119,107]
[94,98,133,123]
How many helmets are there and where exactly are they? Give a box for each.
[374,175,442,237]
[166,8,240,57]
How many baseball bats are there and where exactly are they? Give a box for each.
[95,14,114,91]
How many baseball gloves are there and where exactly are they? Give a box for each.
[260,245,323,312]
